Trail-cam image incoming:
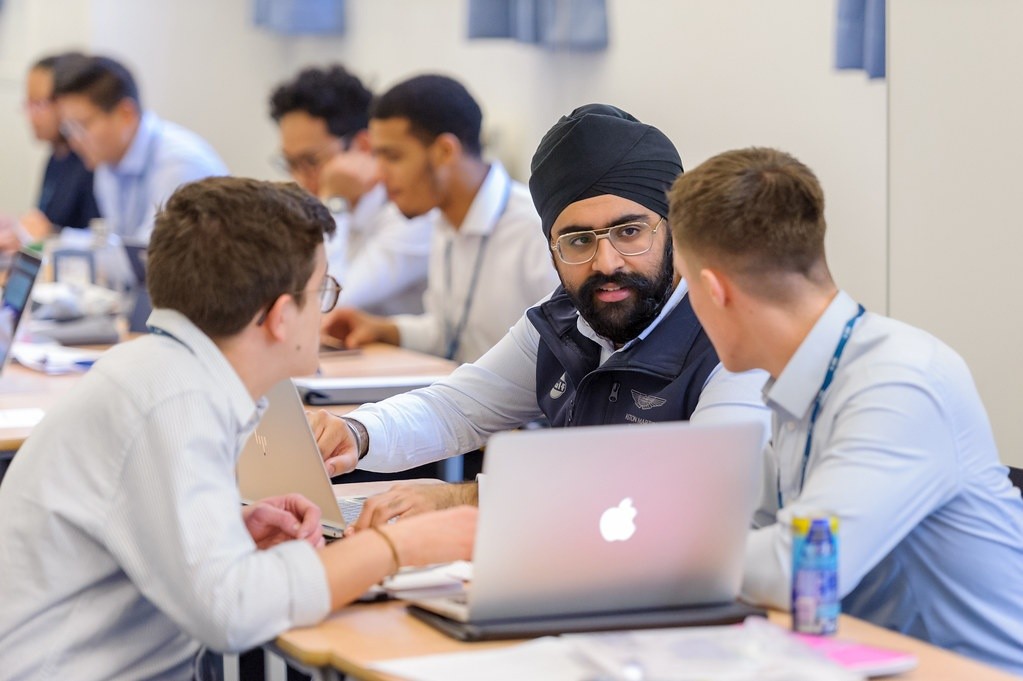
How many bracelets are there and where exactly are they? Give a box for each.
[367,519,400,580]
[347,420,361,460]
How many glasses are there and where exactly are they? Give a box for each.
[550,217,665,264]
[257,275,342,326]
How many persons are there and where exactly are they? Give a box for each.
[662,146,1023,673]
[0,176,476,681]
[0,51,775,538]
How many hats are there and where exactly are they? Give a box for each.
[529,103,684,241]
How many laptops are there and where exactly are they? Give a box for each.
[125,244,165,336]
[229,376,374,539]
[391,420,769,623]
[0,249,42,375]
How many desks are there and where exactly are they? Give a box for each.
[0,273,469,485]
[240,478,1020,681]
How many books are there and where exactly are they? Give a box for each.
[789,628,917,677]
